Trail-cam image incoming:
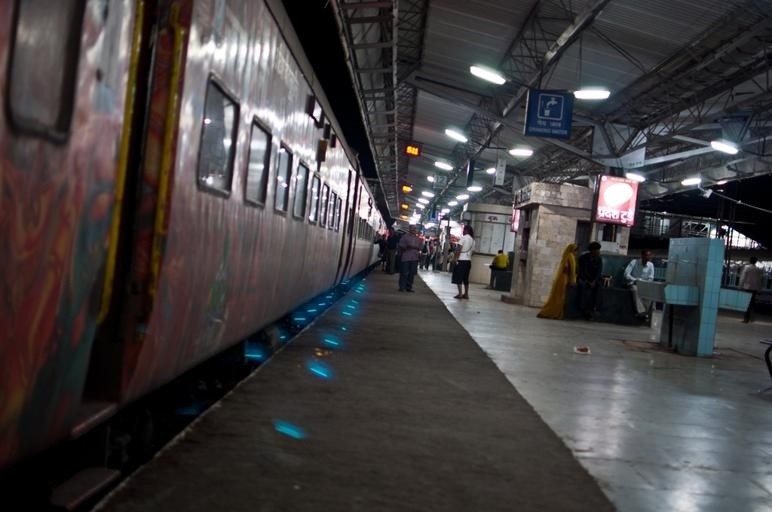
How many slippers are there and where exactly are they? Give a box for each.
[455,294,468,299]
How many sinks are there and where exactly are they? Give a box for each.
[718,288,753,312]
[637,278,699,307]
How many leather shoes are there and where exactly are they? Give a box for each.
[399,288,415,292]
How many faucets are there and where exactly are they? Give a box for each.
[661,258,678,265]
[676,258,697,264]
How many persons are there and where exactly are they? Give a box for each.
[624,249,655,320]
[738,257,762,324]
[489,249,511,289]
[397,225,421,292]
[418,237,458,272]
[451,225,477,300]
[536,243,579,319]
[577,242,605,287]
[369,225,401,274]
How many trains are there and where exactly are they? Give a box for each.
[0,0,387,469]
